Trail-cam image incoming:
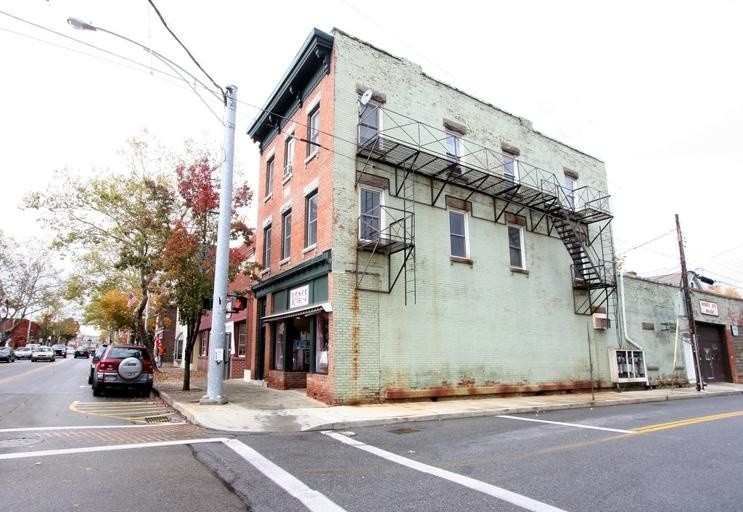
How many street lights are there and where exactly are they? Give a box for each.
[67,15,239,405]
[678,269,714,390]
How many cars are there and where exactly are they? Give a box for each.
[0,342,154,398]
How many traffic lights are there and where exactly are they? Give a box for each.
[231,296,247,311]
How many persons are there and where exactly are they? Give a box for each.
[155,343,167,369]
